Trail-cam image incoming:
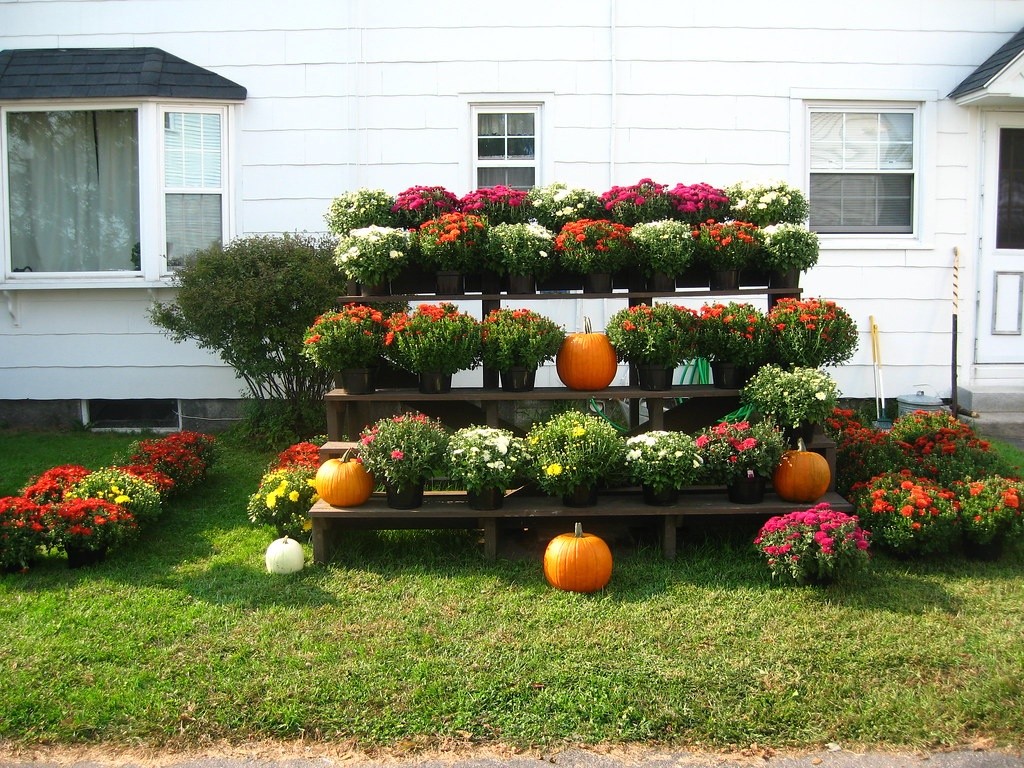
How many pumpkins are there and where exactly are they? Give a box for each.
[264,534,305,575]
[544,523,614,594]
[773,438,832,504]
[555,318,619,391]
[314,447,373,506]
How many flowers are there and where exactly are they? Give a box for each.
[553,218,636,274]
[328,188,404,227]
[456,183,536,227]
[1,498,43,571]
[115,464,175,499]
[665,182,730,227]
[275,442,321,472]
[849,466,960,557]
[40,498,144,550]
[300,303,392,371]
[517,404,626,497]
[440,421,526,495]
[693,418,791,479]
[629,218,699,270]
[523,184,598,231]
[726,182,813,229]
[753,500,873,584]
[377,303,487,376]
[333,224,417,284]
[693,217,763,269]
[415,211,488,269]
[111,439,209,497]
[598,176,674,231]
[349,410,448,490]
[693,302,779,363]
[159,431,222,473]
[758,224,819,271]
[391,182,458,227]
[621,426,704,489]
[16,461,92,503]
[476,306,565,371]
[768,297,860,371]
[63,467,163,524]
[947,472,1024,547]
[246,468,319,535]
[740,362,839,426]
[608,303,702,369]
[485,218,560,273]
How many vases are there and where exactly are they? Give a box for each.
[634,359,675,392]
[275,522,310,546]
[493,359,537,392]
[384,478,427,510]
[466,487,505,513]
[712,357,759,390]
[559,482,600,510]
[723,469,768,502]
[766,268,804,289]
[413,367,452,394]
[961,527,1007,563]
[642,481,680,506]
[646,268,678,292]
[707,266,744,293]
[508,269,537,295]
[357,278,388,299]
[337,366,379,397]
[62,541,106,570]
[433,268,466,297]
[784,420,817,447]
[579,271,615,295]
[877,535,932,563]
